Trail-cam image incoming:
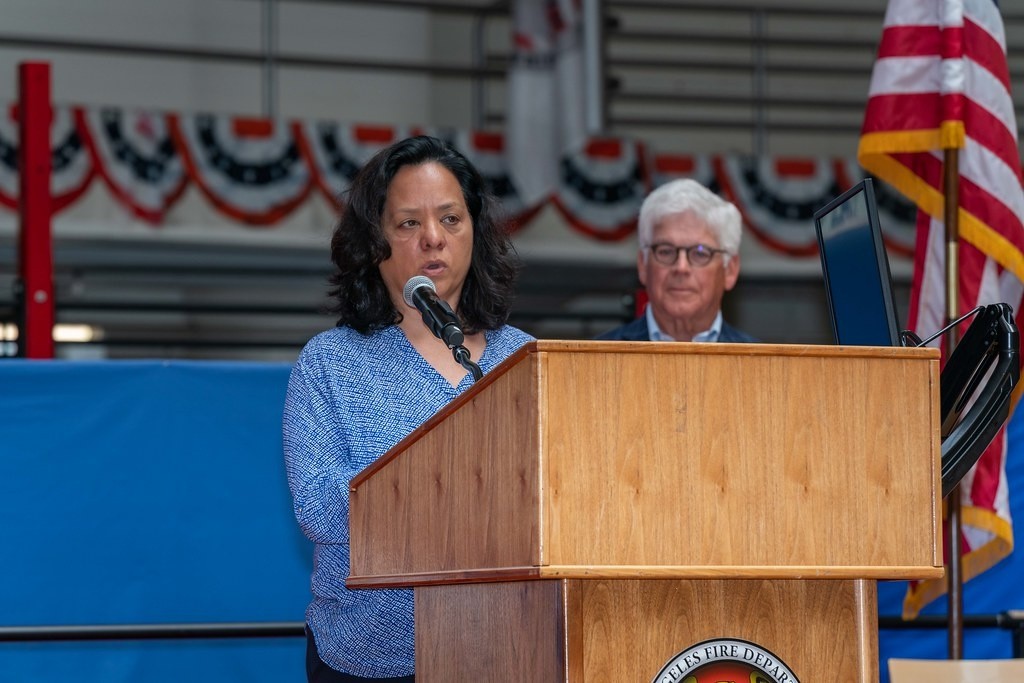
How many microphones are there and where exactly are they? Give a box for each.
[403,275,464,346]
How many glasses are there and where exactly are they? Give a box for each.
[644,241,729,268]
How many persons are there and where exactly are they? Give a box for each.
[283,137,539,683]
[590,179,760,344]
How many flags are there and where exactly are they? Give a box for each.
[858,1,1024,620]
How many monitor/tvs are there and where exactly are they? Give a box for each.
[812,178,903,346]
